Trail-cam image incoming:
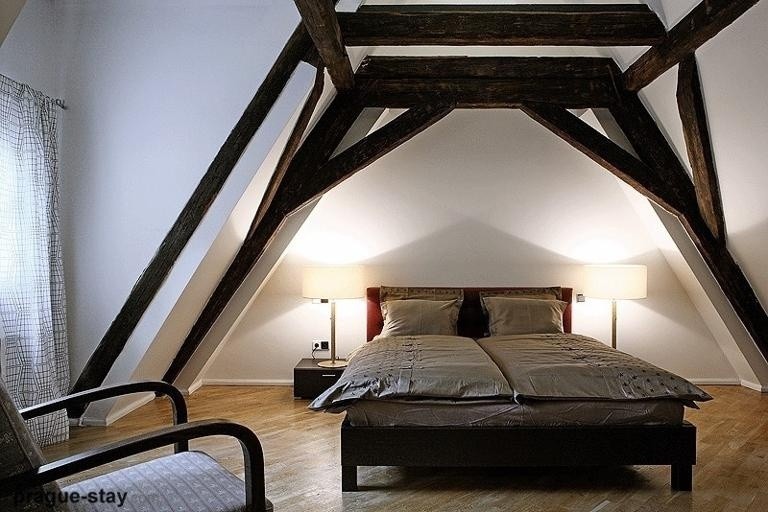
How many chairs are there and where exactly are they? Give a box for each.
[0,375,275,511]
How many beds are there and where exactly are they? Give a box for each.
[341,288,697,492]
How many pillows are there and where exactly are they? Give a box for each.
[373,284,569,339]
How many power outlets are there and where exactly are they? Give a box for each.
[312,340,329,350]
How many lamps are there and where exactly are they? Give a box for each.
[581,262,647,351]
[301,263,366,367]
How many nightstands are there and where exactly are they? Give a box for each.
[294,358,349,400]
